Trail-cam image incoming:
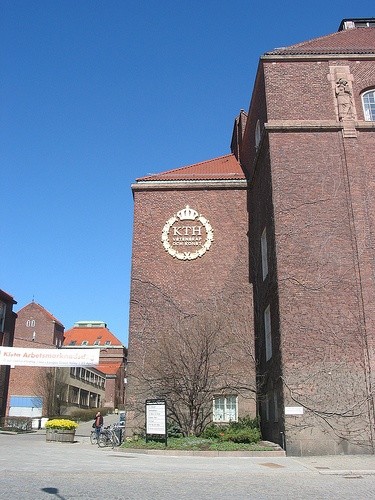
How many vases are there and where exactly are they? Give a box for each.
[45,427,75,443]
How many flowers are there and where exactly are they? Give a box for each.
[44,418,77,430]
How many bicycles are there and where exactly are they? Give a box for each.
[91,424,119,448]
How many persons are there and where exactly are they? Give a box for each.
[94,411,103,440]
